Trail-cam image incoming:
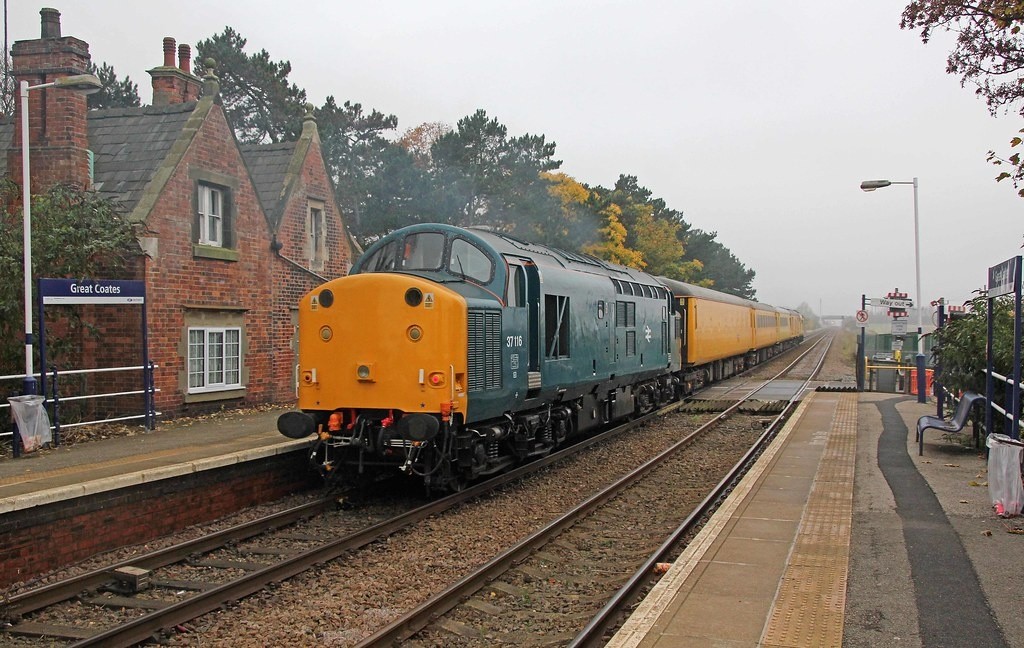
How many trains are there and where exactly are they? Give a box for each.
[274,223,805,499]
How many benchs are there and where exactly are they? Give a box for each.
[916,391,986,456]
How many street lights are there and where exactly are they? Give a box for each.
[860,176,929,404]
[19,72,102,399]
